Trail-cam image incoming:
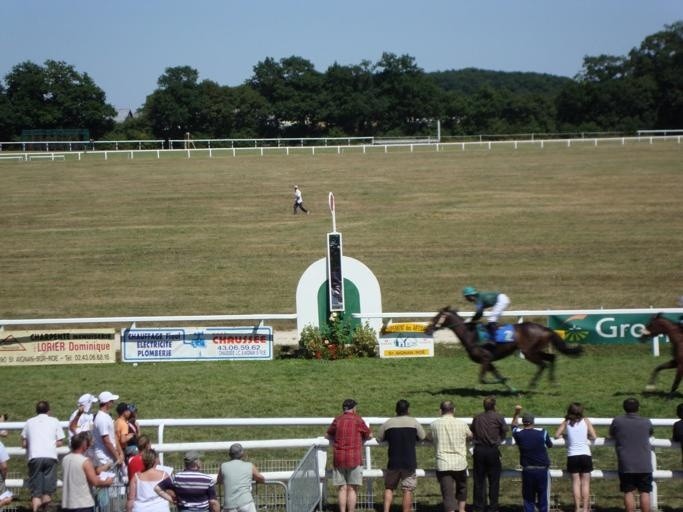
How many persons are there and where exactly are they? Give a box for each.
[293,185,309,215]
[327,399,372,512]
[1,391,265,512]
[463,286,510,345]
[609,398,654,512]
[555,402,596,512]
[427,401,473,512]
[375,399,427,512]
[470,398,510,512]
[673,403,683,453]
[511,405,553,512]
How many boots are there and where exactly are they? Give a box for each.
[488,322,496,344]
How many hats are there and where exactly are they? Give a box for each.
[183,451,199,464]
[99,391,120,403]
[522,413,534,425]
[78,393,98,406]
[117,402,127,415]
[342,399,357,410]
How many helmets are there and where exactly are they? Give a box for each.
[462,287,479,296]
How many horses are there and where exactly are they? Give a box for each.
[638,312,683,393]
[423,305,585,395]
